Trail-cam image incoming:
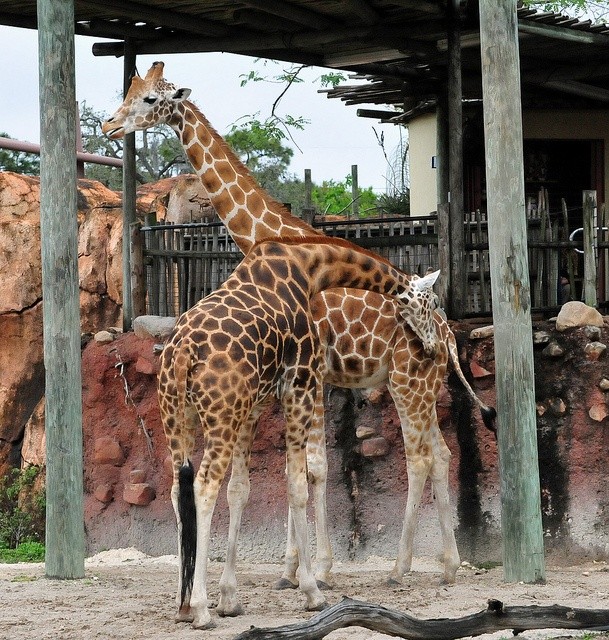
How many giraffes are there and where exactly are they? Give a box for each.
[101,61,497,590]
[156,237,441,630]
